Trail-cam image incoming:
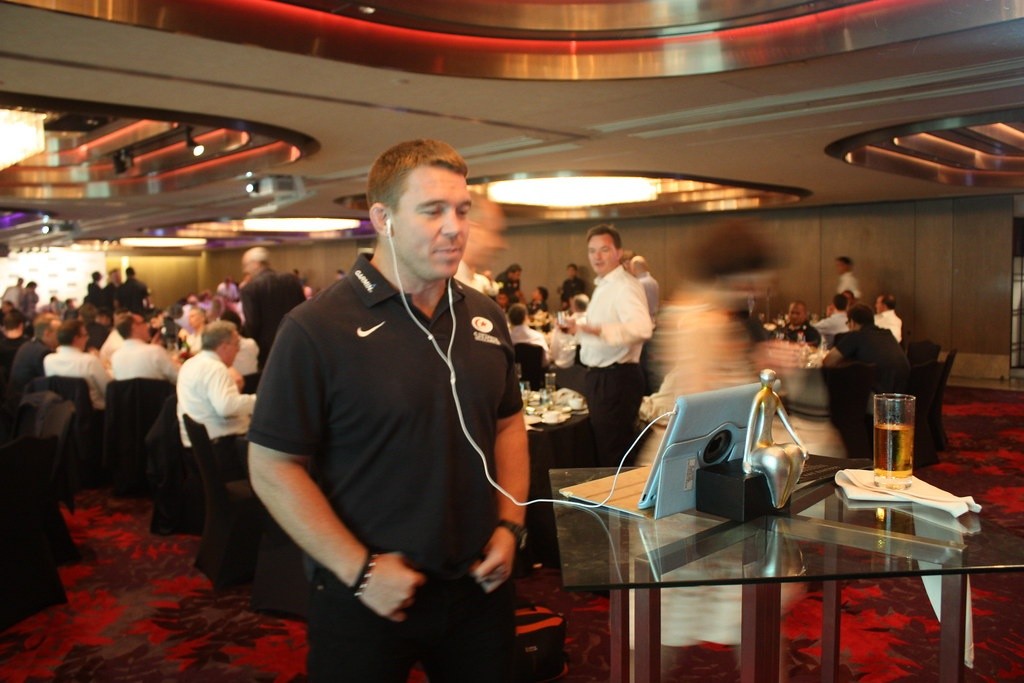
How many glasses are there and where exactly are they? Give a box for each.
[845,320,850,327]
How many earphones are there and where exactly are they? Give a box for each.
[385,219,393,237]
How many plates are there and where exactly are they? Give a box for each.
[541,419,565,425]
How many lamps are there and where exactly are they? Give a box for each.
[184,125,205,157]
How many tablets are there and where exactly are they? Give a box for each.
[636,378,782,510]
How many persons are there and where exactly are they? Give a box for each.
[874,293,902,343]
[836,256,861,299]
[168,275,243,319]
[555,225,652,467]
[822,303,913,459]
[620,250,659,394]
[743,519,806,578]
[811,290,854,349]
[464,257,589,373]
[87,267,151,315]
[291,268,305,287]
[0,296,256,476]
[0,277,38,318]
[240,247,307,381]
[742,369,809,508]
[783,300,821,348]
[245,140,534,683]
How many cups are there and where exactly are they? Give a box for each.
[873,393,915,490]
[542,411,561,423]
[557,311,569,333]
[540,389,549,411]
[570,398,584,410]
[527,407,535,415]
[546,373,555,395]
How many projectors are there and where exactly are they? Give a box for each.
[41,224,82,235]
[246,175,304,198]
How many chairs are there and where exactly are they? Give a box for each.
[529,415,606,568]
[0,375,262,633]
[821,337,958,470]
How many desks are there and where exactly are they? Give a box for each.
[549,467,1024,683]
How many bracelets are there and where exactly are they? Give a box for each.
[349,552,376,594]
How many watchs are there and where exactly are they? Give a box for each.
[496,519,527,551]
